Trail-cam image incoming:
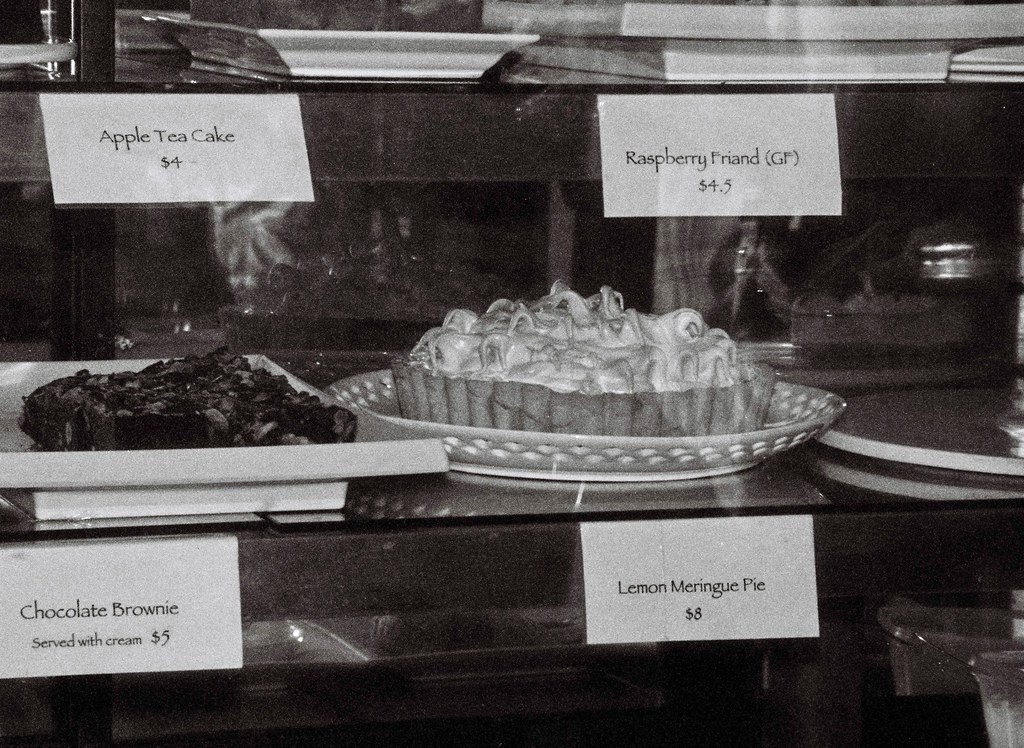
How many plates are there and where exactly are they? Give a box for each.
[328,371,851,487]
[0,353,454,530]
[145,15,540,90]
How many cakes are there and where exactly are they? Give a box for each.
[391,280,776,439]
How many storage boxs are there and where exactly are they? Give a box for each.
[3,26,1022,681]
